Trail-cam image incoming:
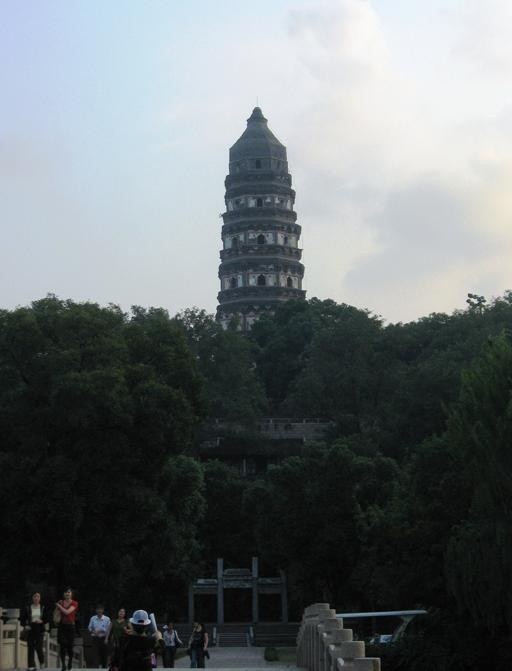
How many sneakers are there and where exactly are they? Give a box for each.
[68,662,72,670]
[40,661,45,669]
[61,665,66,671]
[27,667,36,671]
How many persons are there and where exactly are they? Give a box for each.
[162,619,184,668]
[20,590,49,671]
[188,621,209,669]
[52,586,79,671]
[110,609,159,671]
[87,602,111,669]
[104,604,133,667]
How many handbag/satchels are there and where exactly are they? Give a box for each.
[20,626,31,641]
[52,601,62,625]
[173,630,185,648]
[203,650,210,659]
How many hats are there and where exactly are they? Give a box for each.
[159,623,169,629]
[192,623,199,627]
[128,609,152,626]
